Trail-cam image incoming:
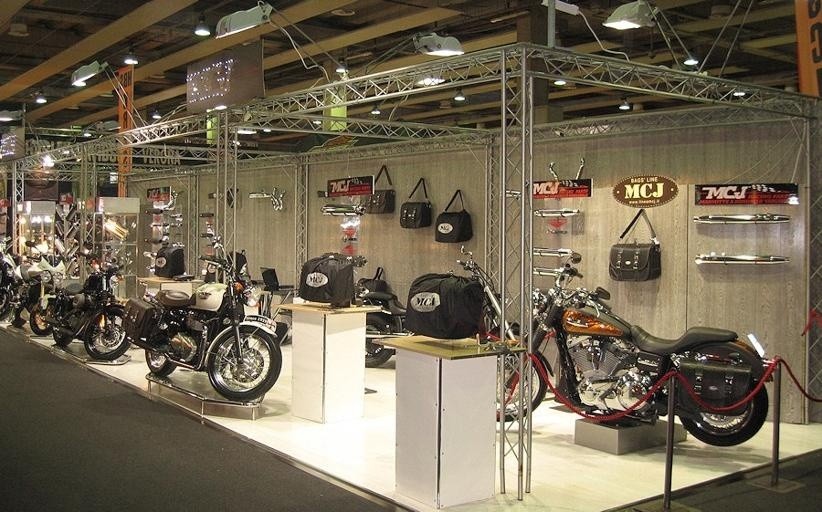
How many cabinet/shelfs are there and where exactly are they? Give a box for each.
[78,195,142,296]
[372,334,527,509]
[695,220,786,266]
[18,200,57,266]
[142,277,205,299]
[281,304,383,421]
[530,208,578,276]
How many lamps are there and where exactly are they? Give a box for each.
[152,108,161,119]
[336,61,346,73]
[413,31,464,57]
[71,60,102,85]
[263,120,271,132]
[683,58,699,65]
[215,4,273,39]
[371,102,380,114]
[194,13,211,36]
[453,88,466,101]
[124,48,139,64]
[602,0,651,31]
[36,91,47,104]
[619,95,632,111]
[230,122,257,134]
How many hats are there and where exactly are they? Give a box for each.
[357,267,387,293]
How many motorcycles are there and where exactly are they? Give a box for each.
[1,227,283,401]
[498,253,774,447]
[353,245,520,367]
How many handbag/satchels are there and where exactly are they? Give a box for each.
[204,263,222,282]
[365,165,396,214]
[400,178,432,228]
[299,252,353,306]
[404,273,484,339]
[154,246,185,278]
[227,251,247,277]
[122,297,154,341]
[609,208,661,282]
[435,189,472,242]
[677,359,752,416]
